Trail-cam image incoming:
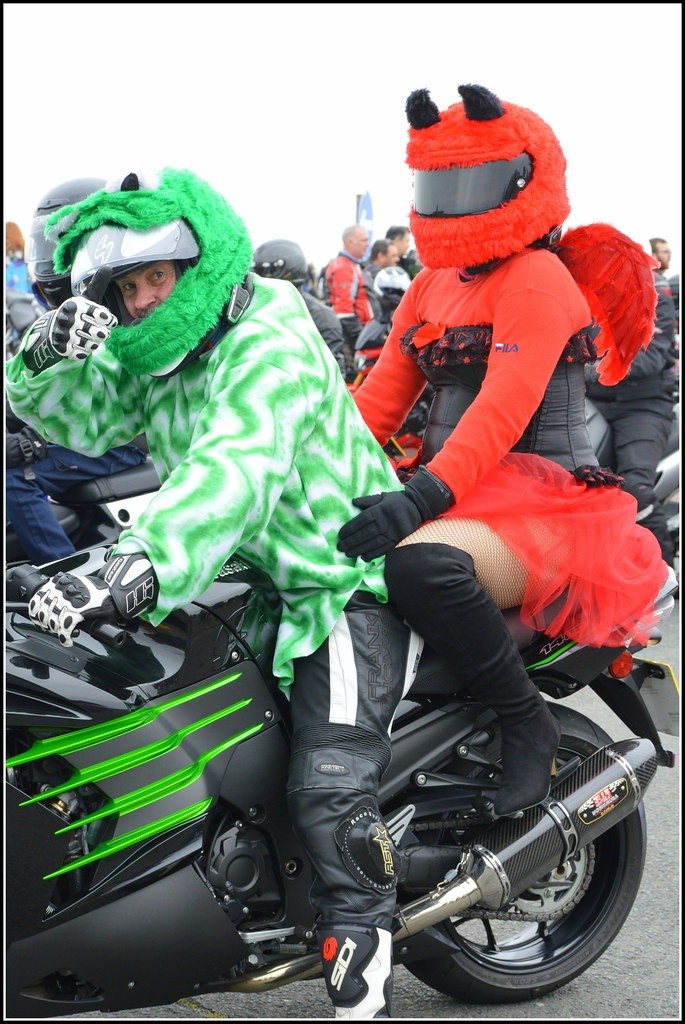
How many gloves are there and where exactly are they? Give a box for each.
[28,551,160,649]
[22,265,118,371]
[5,425,48,468]
[337,465,456,563]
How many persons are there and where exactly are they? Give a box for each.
[3,166,425,1018]
[252,225,417,382]
[336,85,668,815]
[7,179,150,564]
[586,238,678,568]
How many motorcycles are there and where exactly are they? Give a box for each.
[6,282,680,1018]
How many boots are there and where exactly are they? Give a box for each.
[385,544,560,815]
[314,920,393,1019]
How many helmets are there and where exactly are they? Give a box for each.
[28,177,110,311]
[252,239,305,287]
[374,265,411,308]
[70,217,204,376]
[410,153,563,284]
